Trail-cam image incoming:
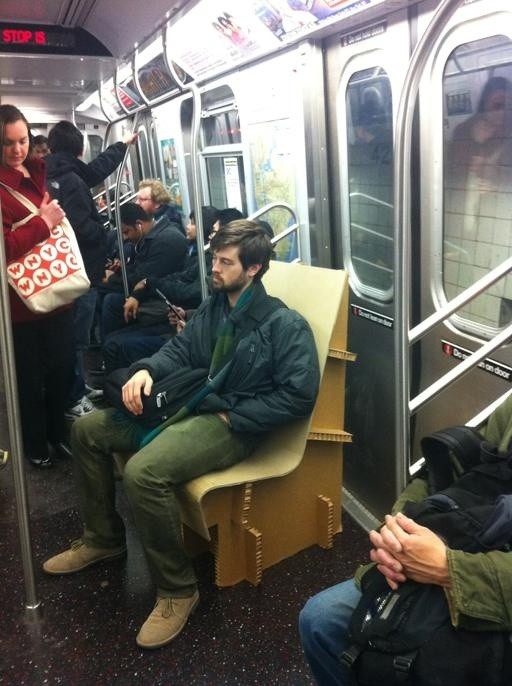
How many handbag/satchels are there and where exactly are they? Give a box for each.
[133,297,170,328]
[102,366,209,429]
[7,200,91,318]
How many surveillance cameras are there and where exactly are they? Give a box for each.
[94,123,98,129]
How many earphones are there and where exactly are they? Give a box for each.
[139,224,142,227]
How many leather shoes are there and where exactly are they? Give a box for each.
[29,441,75,472]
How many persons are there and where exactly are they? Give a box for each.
[350,77,512,305]
[297,386,511,686]
[2,103,277,482]
[42,216,322,651]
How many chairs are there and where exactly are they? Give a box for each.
[110,260,357,588]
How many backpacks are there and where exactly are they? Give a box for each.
[338,423,511,686]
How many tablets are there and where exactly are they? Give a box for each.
[155,288,189,324]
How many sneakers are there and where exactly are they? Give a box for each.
[43,538,124,576]
[136,590,201,651]
[63,384,102,424]
